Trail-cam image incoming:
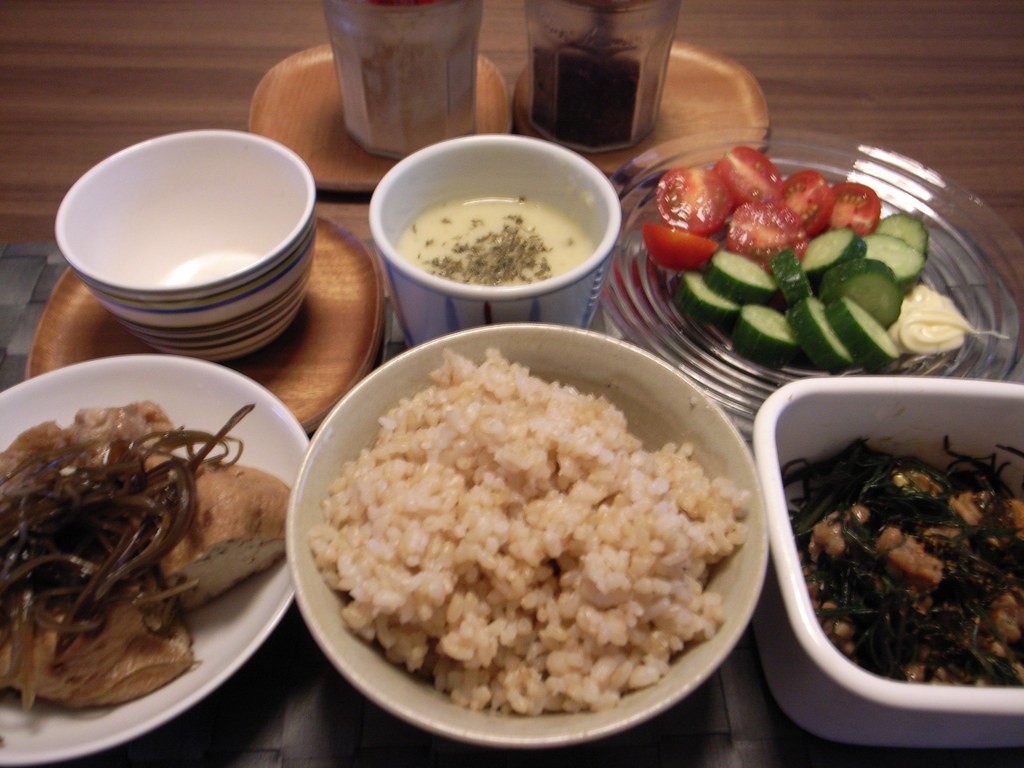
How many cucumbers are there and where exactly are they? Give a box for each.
[672,211,930,374]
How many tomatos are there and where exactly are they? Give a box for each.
[640,146,882,274]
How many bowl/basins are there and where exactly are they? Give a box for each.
[753,375,1023,750]
[54,129,318,367]
[284,323,770,750]
[368,133,623,348]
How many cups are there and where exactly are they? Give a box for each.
[526,0,684,151]
[323,0,485,160]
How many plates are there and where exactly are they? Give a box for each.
[0,354,310,768]
[601,130,1024,443]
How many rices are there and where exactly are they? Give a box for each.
[312,342,749,721]
